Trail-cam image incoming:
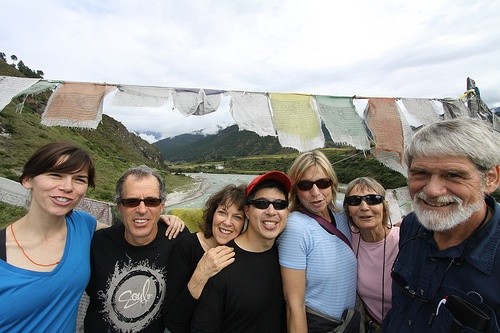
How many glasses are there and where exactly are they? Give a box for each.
[345,193,386,206]
[120,196,164,209]
[296,177,334,191]
[246,197,290,210]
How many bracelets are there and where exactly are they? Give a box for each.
[393,224,396,227]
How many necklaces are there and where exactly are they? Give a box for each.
[355,225,386,326]
[11,223,59,266]
[390,206,489,304]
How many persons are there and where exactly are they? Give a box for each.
[83,165,191,333]
[342,177,400,333]
[163,184,248,333]
[0,143,185,333]
[185,169,292,333]
[379,115,500,333]
[275,150,402,333]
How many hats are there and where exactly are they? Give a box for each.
[245,171,292,196]
